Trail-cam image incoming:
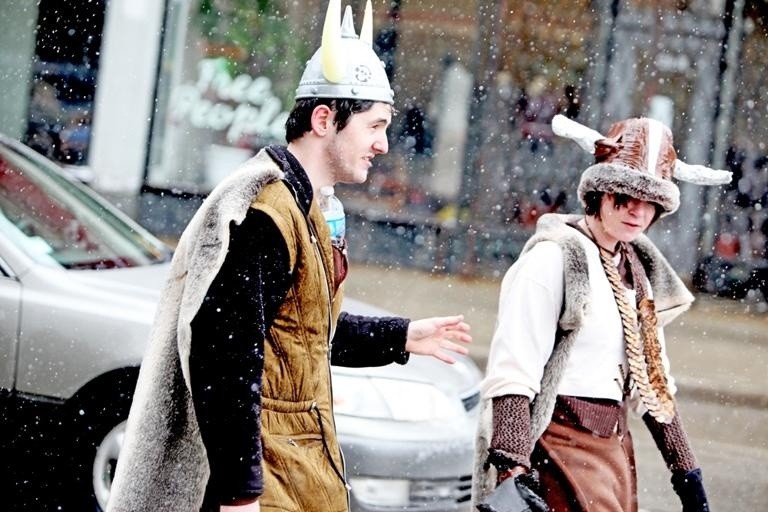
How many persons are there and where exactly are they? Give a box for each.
[481,191,712,511]
[104,98,472,512]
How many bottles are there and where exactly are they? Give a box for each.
[320,184,345,247]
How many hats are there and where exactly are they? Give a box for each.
[577,118,680,218]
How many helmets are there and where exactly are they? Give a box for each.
[295,36,394,104]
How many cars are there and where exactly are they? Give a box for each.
[0,131,483,510]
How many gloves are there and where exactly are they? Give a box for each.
[671,468,710,512]
